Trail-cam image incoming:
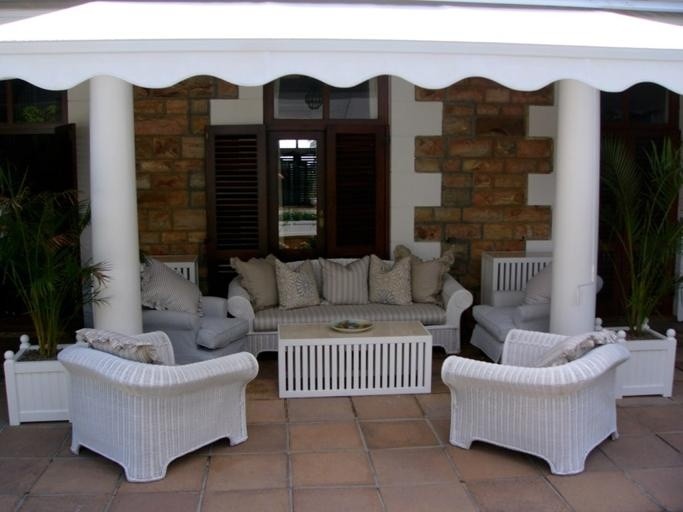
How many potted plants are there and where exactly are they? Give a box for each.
[0,158,114,426]
[598,135,683,400]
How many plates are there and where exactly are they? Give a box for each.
[330,319,372,332]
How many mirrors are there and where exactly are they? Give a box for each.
[270,134,321,239]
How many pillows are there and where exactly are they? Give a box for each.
[393,245,450,309]
[230,256,279,313]
[369,254,412,306]
[275,258,321,311]
[523,261,604,305]
[319,255,370,305]
[528,330,619,369]
[140,255,204,317]
[75,327,163,364]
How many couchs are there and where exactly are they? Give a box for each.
[228,244,474,356]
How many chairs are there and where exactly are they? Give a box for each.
[141,259,250,374]
[57,327,260,483]
[441,329,631,475]
[469,261,604,366]
